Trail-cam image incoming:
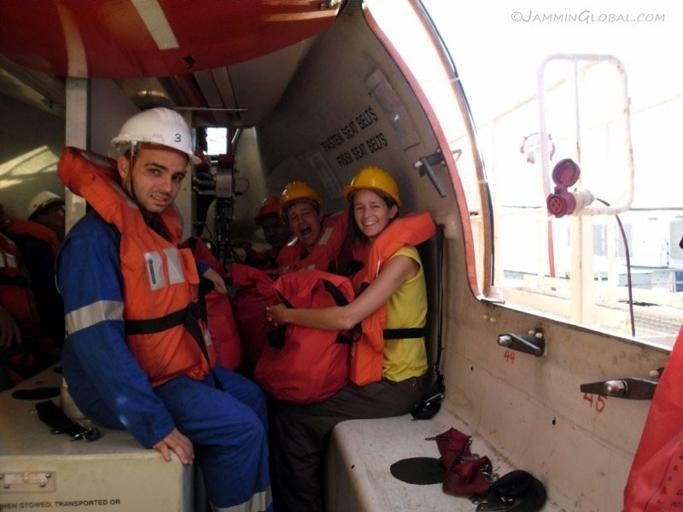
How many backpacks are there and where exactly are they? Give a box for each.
[252,194,286,270]
[272,165,430,512]
[254,272,355,405]
[271,180,354,289]
[56,106,273,512]
[18,191,66,354]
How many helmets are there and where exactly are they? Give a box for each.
[342,164,403,211]
[278,179,323,212]
[247,194,282,228]
[109,106,204,166]
[27,189,66,220]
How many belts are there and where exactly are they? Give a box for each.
[32,399,101,442]
